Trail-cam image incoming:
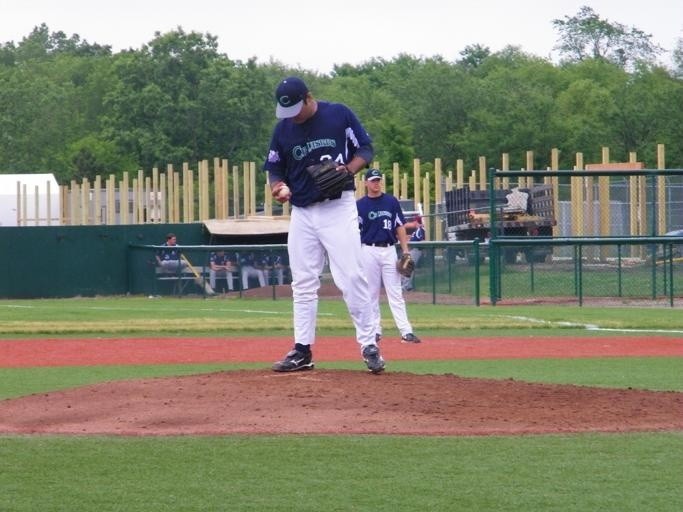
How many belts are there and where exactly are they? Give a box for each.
[366,242,395,247]
[313,192,341,202]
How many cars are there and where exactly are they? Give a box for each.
[657,227,683,255]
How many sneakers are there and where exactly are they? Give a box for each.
[375,334,381,342]
[362,344,385,372]
[401,333,420,343]
[272,349,314,371]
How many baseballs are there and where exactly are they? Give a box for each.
[278,185,291,198]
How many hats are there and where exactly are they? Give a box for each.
[275,78,307,119]
[365,169,382,181]
[412,216,421,223]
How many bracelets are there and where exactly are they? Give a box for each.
[346,166,348,171]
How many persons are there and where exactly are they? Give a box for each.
[356,169,422,344]
[260,245,285,285]
[154,232,188,278]
[401,216,424,292]
[262,77,386,375]
[234,242,266,289]
[208,250,234,291]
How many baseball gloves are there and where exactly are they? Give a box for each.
[304,158,354,196]
[396,253,414,278]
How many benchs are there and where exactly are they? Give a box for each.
[154,266,291,294]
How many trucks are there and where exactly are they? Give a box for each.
[440,180,558,264]
